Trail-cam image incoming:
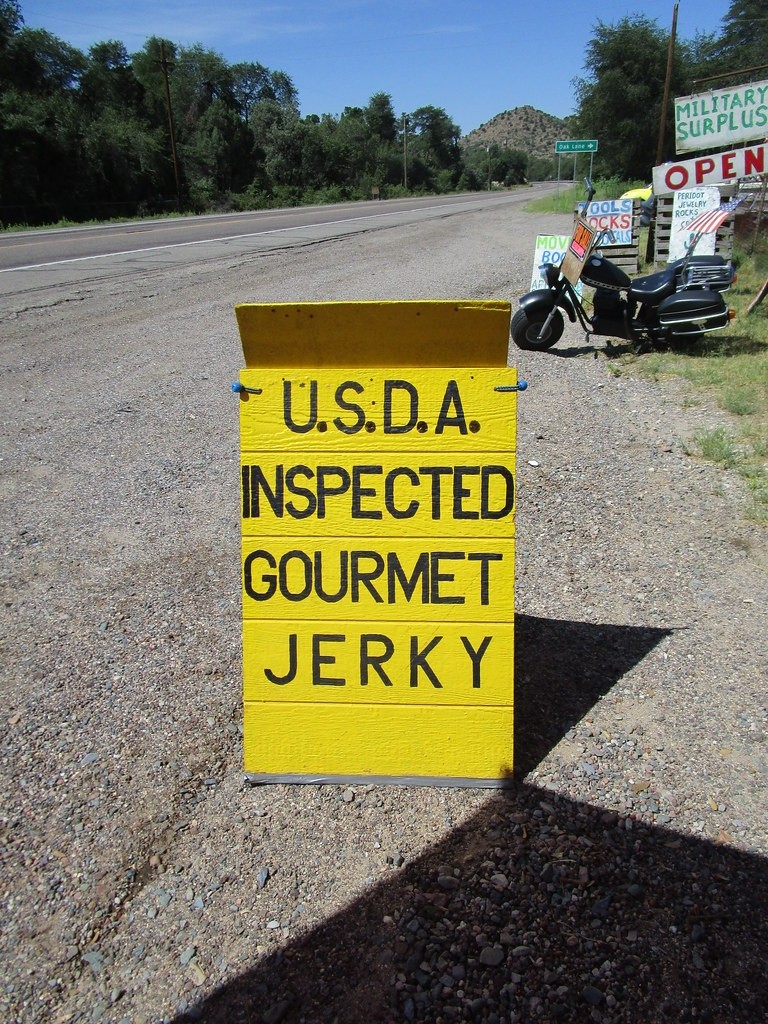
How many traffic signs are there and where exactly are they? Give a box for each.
[555,139,598,153]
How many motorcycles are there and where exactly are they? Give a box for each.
[510,176,755,358]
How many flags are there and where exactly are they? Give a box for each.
[677,196,748,234]
